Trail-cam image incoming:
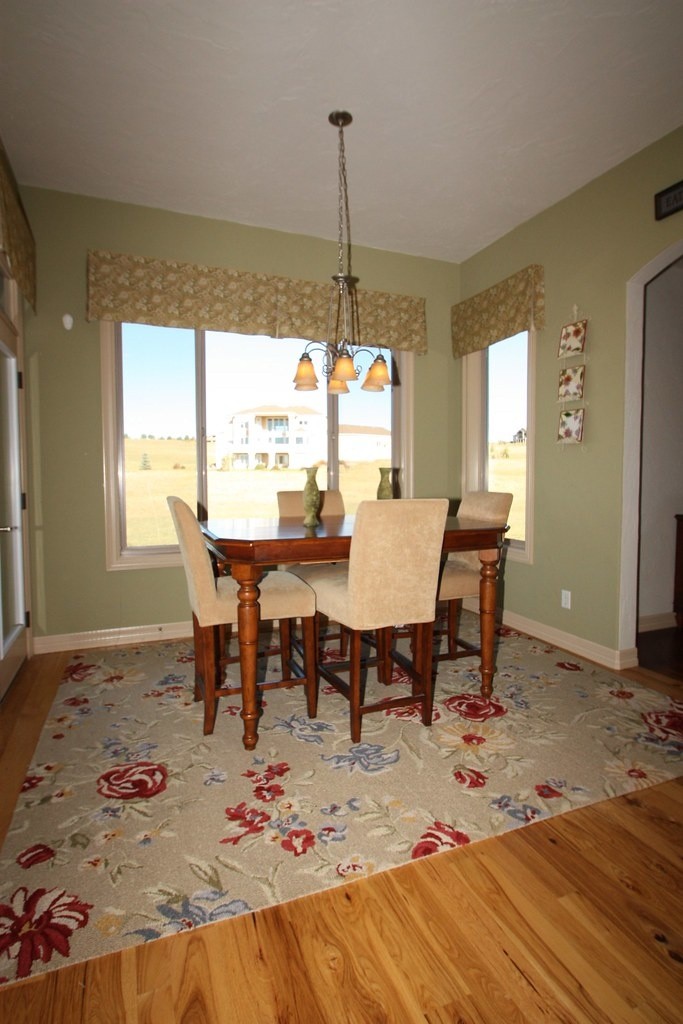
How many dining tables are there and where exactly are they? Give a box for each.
[198,515,510,751]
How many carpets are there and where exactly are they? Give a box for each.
[0,597,683,989]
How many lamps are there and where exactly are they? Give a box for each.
[293,111,392,394]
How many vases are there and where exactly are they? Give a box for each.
[391,468,402,498]
[301,466,323,529]
[377,468,393,499]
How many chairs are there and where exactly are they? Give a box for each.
[168,497,317,751]
[287,498,450,743]
[277,490,347,659]
[375,493,515,696]
